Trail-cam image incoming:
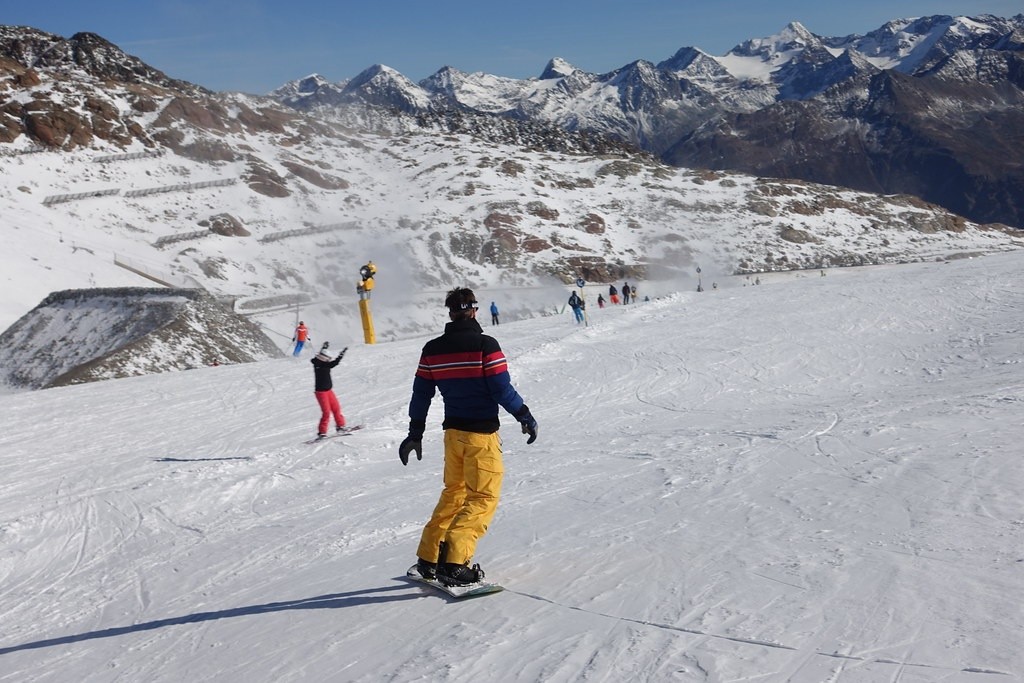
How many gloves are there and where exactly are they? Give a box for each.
[293,338,295,341]
[308,338,310,341]
[399,436,422,465]
[521,412,538,444]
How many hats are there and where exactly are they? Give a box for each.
[321,342,333,358]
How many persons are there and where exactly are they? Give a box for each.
[608,279,652,306]
[490,301,500,326]
[397,287,540,586]
[292,321,311,357]
[309,341,349,439]
[568,290,584,324]
[597,293,607,309]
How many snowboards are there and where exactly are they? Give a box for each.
[408,563,504,598]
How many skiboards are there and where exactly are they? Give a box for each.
[303,424,365,444]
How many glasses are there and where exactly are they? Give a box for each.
[475,302,479,311]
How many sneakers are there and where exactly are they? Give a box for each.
[318,433,328,439]
[336,425,351,432]
[437,562,485,587]
[417,558,437,579]
